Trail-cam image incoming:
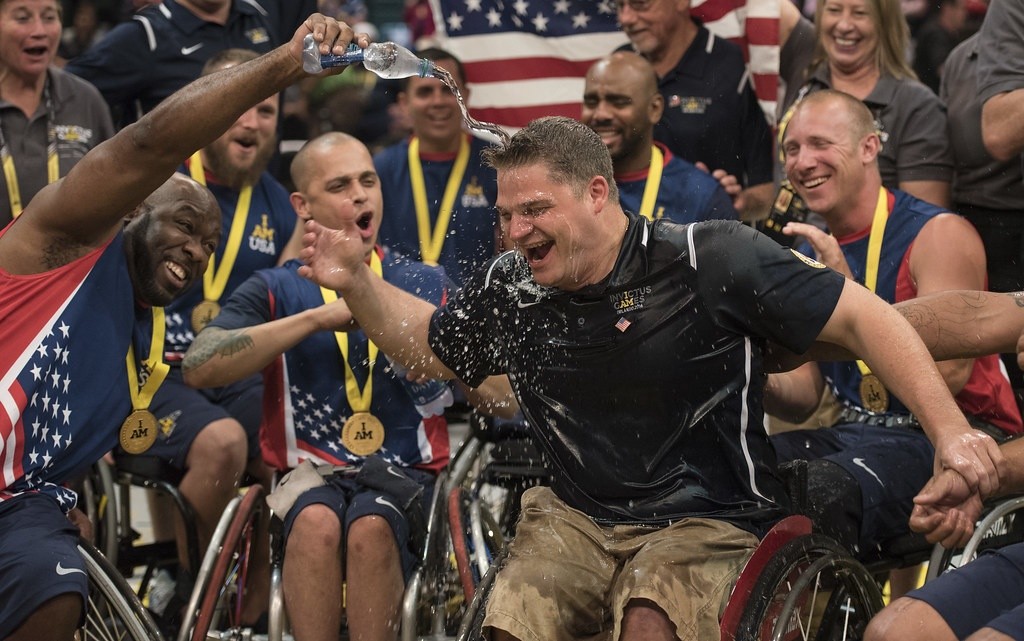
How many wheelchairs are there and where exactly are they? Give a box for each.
[54,352,1024,640]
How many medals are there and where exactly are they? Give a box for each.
[859,374,888,412]
[120,410,158,455]
[341,413,385,456]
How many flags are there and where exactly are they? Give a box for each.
[428,0,780,147]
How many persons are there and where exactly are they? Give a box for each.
[578,50,740,223]
[0,14,370,641]
[919,0,1024,415]
[0,1,115,231]
[373,48,505,286]
[181,131,520,641]
[780,0,951,211]
[863,332,1024,641]
[62,0,279,125]
[615,0,774,234]
[760,90,1022,570]
[296,117,1009,641]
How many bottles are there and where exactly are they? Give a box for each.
[300,31,436,81]
